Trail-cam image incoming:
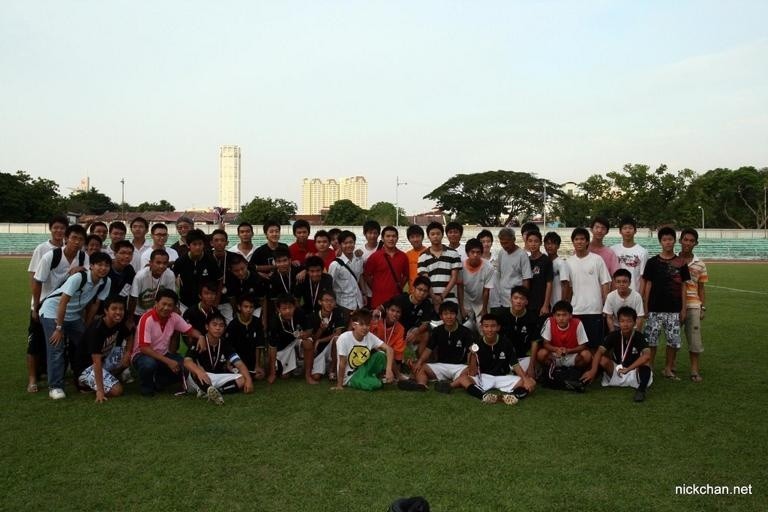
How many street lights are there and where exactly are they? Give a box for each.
[396,177,408,228]
[698,205,706,229]
[542,179,548,231]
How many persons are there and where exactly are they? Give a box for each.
[26,216,709,406]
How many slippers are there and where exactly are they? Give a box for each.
[690,374,702,383]
[663,374,680,381]
[27,384,39,393]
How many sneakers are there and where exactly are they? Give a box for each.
[49,388,66,400]
[482,394,498,405]
[195,388,206,400]
[399,381,426,391]
[434,382,452,393]
[499,394,519,406]
[207,386,225,408]
[635,390,644,401]
